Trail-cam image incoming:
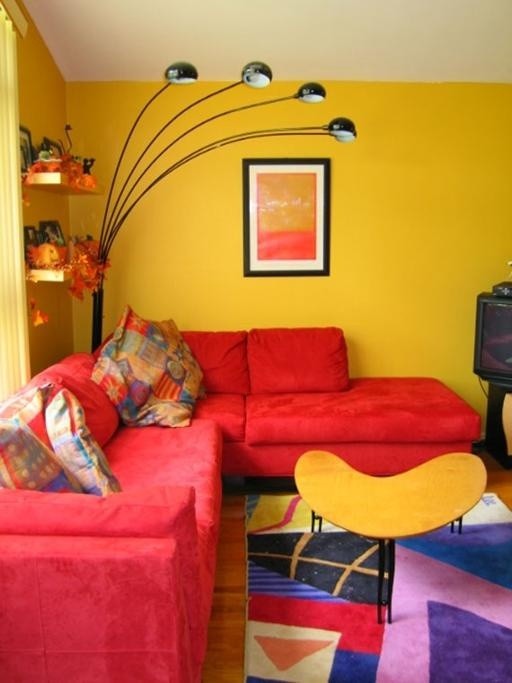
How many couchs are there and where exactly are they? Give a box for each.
[91,326,482,479]
[0,352,225,683]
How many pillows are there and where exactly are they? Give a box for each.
[152,318,207,400]
[0,383,83,493]
[179,328,249,397]
[247,328,350,394]
[89,303,203,428]
[43,374,122,498]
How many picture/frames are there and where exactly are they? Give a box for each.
[242,158,329,277]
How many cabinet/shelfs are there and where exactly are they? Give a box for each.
[473,381,511,470]
[23,171,102,282]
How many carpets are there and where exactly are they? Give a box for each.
[243,493,511,683]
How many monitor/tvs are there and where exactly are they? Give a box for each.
[472,292,512,385]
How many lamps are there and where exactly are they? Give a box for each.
[92,61,358,348]
